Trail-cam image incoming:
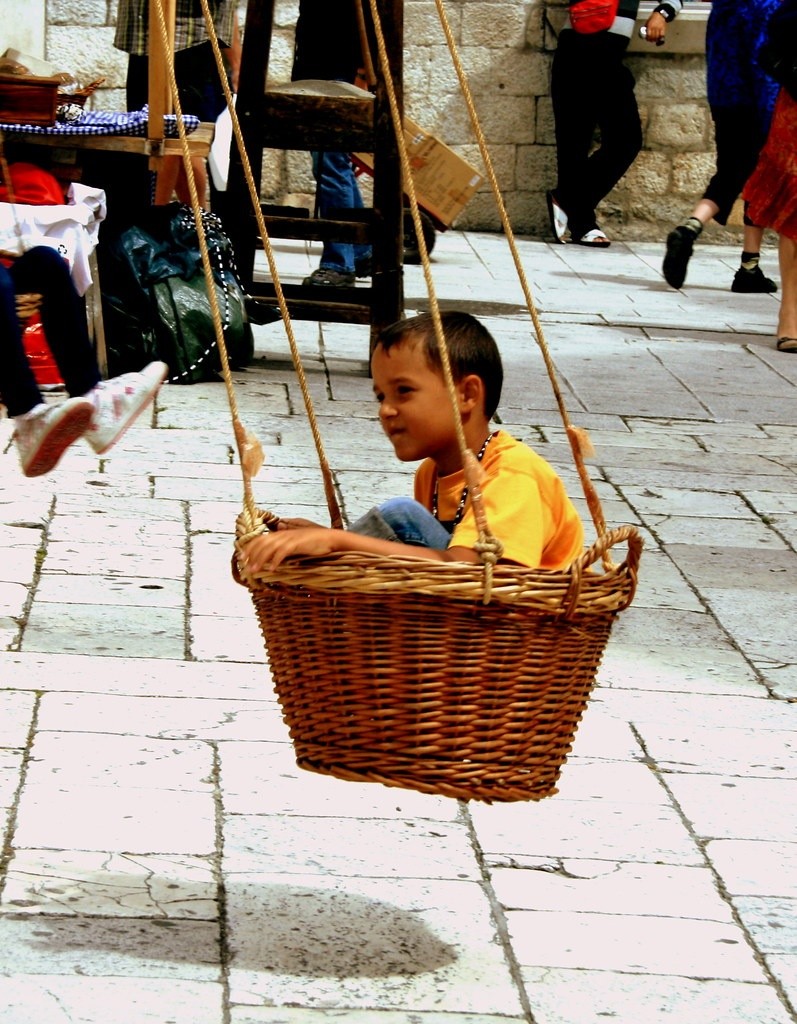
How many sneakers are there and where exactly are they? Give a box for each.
[663,226,697,290]
[310,268,355,288]
[81,361,168,455]
[10,397,96,477]
[354,254,373,279]
[731,264,777,293]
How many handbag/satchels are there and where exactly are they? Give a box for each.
[568,0,619,34]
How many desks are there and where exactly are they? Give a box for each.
[0,118,216,205]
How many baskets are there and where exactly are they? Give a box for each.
[57,78,106,111]
[229,509,645,806]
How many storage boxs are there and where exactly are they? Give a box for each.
[352,114,484,226]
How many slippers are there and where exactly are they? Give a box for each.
[776,337,797,351]
[546,189,568,244]
[570,229,610,248]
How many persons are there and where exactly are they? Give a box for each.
[238,312,585,578]
[663,1,797,351]
[0,246,169,477]
[115,0,377,289]
[539,0,683,246]
